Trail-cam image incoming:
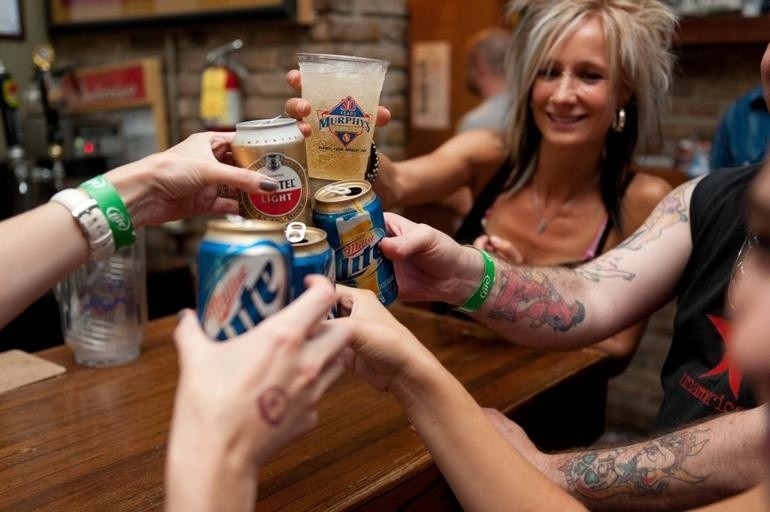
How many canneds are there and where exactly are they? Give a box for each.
[194,212,294,341]
[231,113,312,227]
[280,221,340,321]
[311,178,399,306]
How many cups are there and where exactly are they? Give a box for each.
[48,224,148,369]
[294,52,392,183]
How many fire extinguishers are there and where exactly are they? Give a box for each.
[196,39,248,132]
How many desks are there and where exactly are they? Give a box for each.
[0,304,611,511]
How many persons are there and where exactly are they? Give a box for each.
[283,0,768,512]
[0,126,354,511]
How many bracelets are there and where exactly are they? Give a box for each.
[80,174,136,255]
[48,186,115,262]
